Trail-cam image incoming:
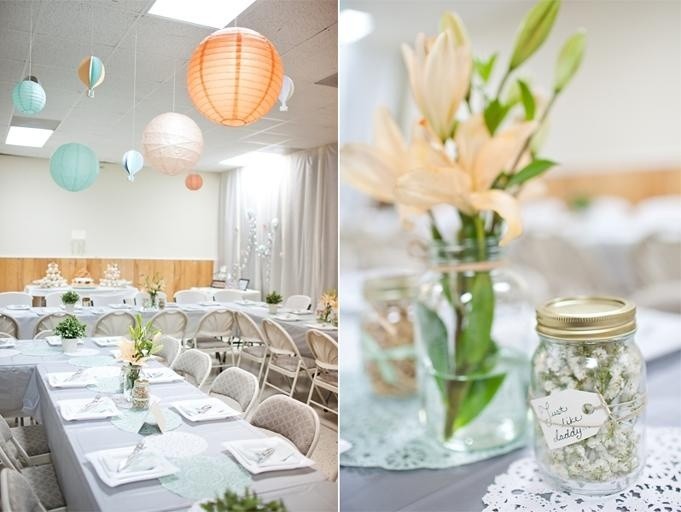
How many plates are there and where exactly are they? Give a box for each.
[6,298,338,331]
[0,335,317,487]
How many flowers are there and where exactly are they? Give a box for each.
[339,0,590,441]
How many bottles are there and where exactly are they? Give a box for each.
[529,293,648,498]
[362,270,420,402]
